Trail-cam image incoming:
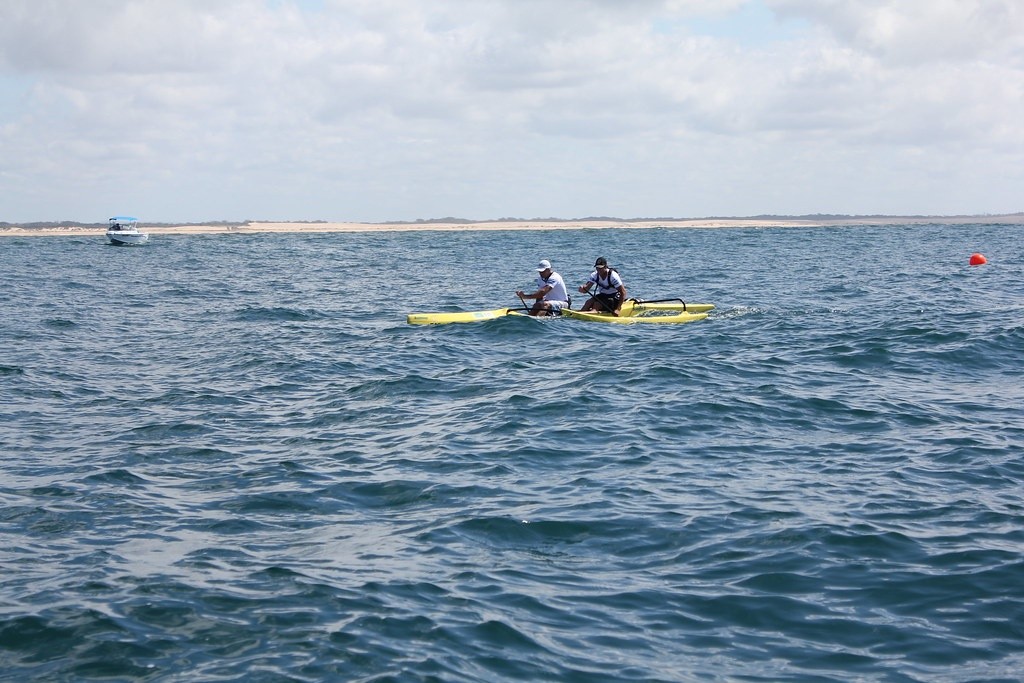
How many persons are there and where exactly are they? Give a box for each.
[516,259,570,317]
[579,258,626,317]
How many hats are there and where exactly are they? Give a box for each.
[593,257,607,268]
[536,259,551,272]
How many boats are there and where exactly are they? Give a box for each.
[106,216,148,246]
[559,297,714,324]
[406,294,571,325]
